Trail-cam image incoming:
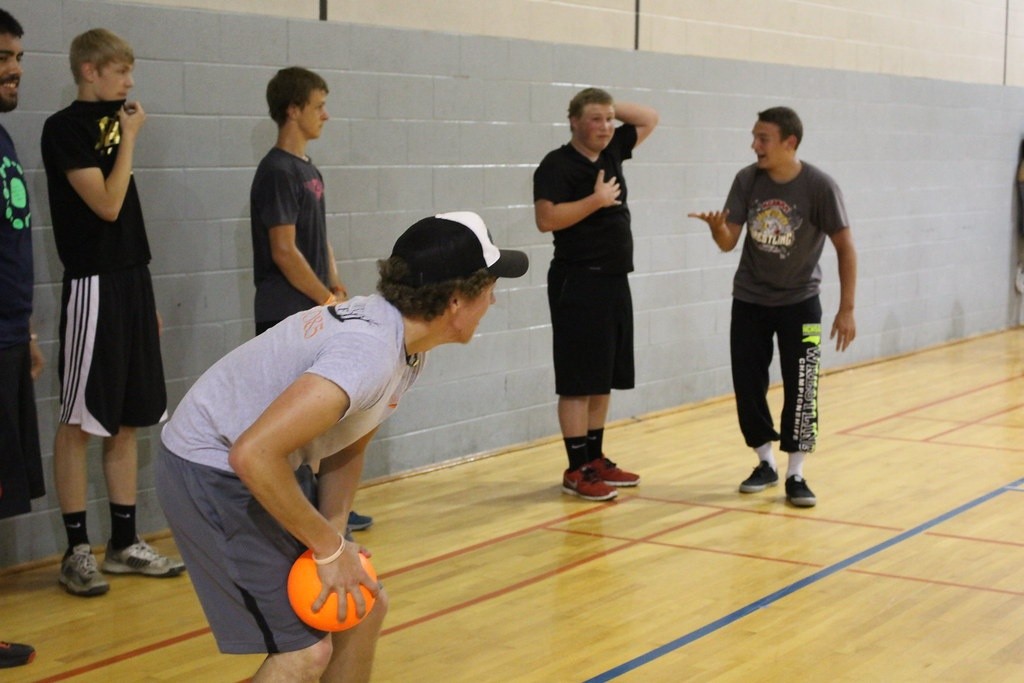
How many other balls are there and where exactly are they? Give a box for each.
[285,543,381,634]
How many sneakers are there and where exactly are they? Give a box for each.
[59,543,109,596]
[562,465,618,501]
[102,533,186,577]
[587,452,640,487]
[740,460,779,493]
[0,641,35,666]
[347,510,372,531]
[785,475,816,506]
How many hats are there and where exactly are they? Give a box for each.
[382,211,528,287]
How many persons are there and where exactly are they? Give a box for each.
[153,212,530,683]
[39,29,186,597]
[533,89,660,499]
[249,67,373,543]
[0,11,46,671]
[688,106,856,507]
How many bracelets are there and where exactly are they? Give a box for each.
[329,287,347,299]
[29,334,37,340]
[325,294,335,308]
[313,533,345,566]
